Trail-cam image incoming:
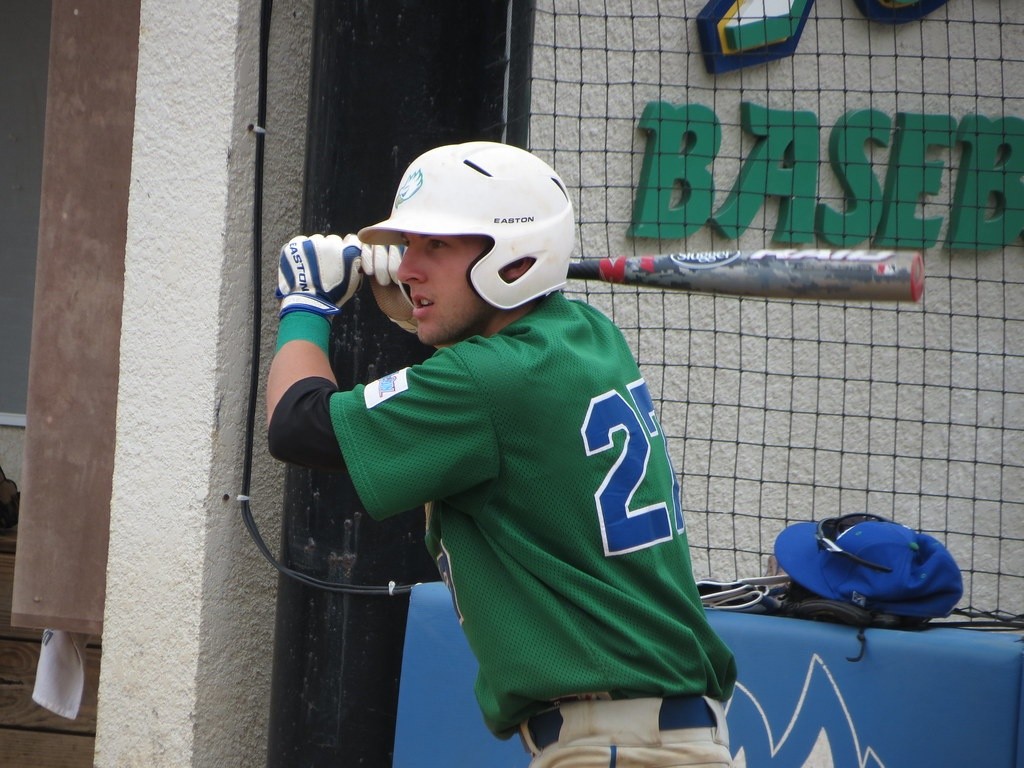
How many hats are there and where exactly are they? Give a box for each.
[773,522,964,618]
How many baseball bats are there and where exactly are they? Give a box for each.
[566,249,926,302]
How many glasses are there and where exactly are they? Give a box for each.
[815,513,917,574]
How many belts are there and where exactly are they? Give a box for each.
[516,695,717,752]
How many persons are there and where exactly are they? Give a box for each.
[268,141,738,768]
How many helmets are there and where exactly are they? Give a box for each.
[357,141,574,310]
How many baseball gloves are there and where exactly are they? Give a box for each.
[781,581,928,632]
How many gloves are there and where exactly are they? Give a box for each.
[275,234,364,330]
[361,241,409,287]
